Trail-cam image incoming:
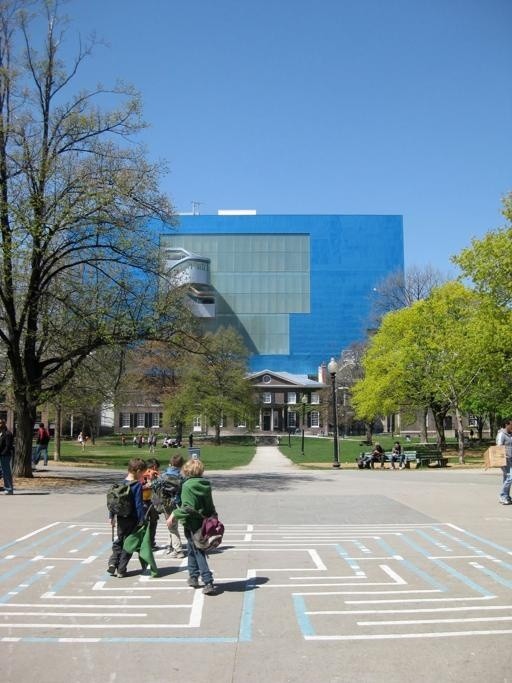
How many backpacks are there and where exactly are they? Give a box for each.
[142,469,159,501]
[160,474,182,508]
[185,508,224,552]
[106,479,139,518]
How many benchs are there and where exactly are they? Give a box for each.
[360,450,449,468]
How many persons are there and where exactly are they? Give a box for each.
[78,432,88,453]
[166,459,218,594]
[158,455,187,558]
[33,423,50,466]
[496,419,512,505]
[371,443,389,470]
[107,458,148,577]
[390,442,405,470]
[470,429,474,439]
[0,418,13,495]
[133,429,193,454]
[139,458,162,552]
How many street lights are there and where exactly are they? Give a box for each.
[300,394,310,457]
[286,405,294,449]
[325,356,344,472]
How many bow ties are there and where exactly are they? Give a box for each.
[187,577,199,588]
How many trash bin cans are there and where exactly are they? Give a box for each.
[188,447,201,460]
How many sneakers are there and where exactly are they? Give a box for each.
[499,495,512,505]
[2,490,13,496]
[107,564,128,578]
[201,583,216,595]
[166,546,185,558]
